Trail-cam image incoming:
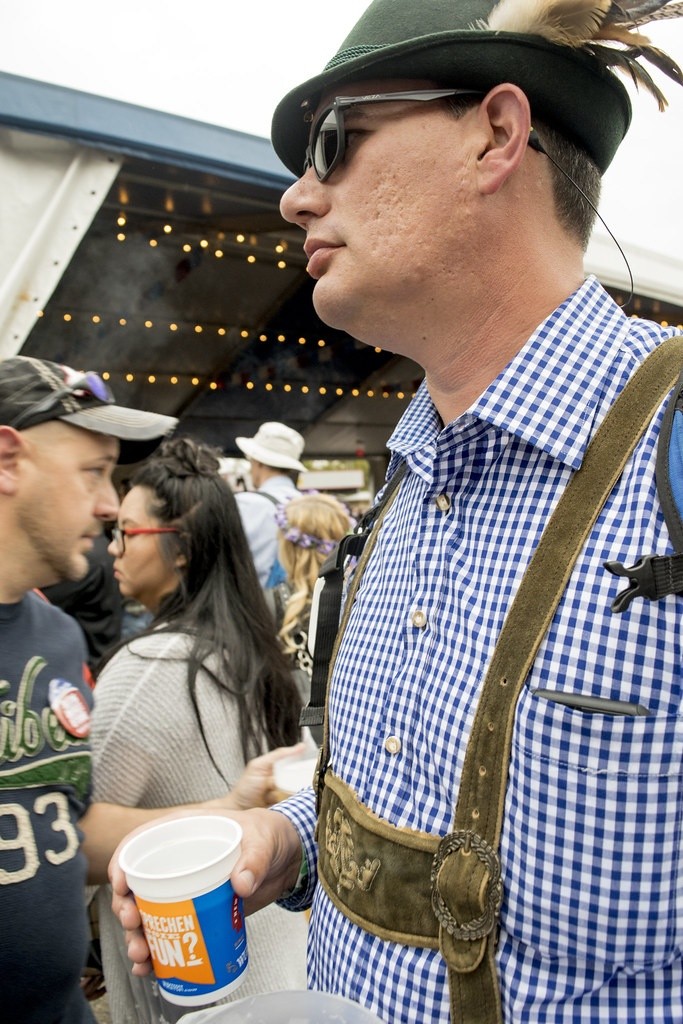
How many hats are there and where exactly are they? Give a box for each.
[236,420,306,473]
[2,354,181,440]
[270,0,681,172]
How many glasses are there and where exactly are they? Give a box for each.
[304,84,544,184]
[13,368,117,425]
[108,527,177,559]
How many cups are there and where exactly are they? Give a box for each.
[118,815,250,1007]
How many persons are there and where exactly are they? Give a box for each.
[39,533,124,682]
[88,438,319,1023]
[109,2,681,1023]
[1,354,308,1023]
[232,422,308,632]
[273,488,355,706]
